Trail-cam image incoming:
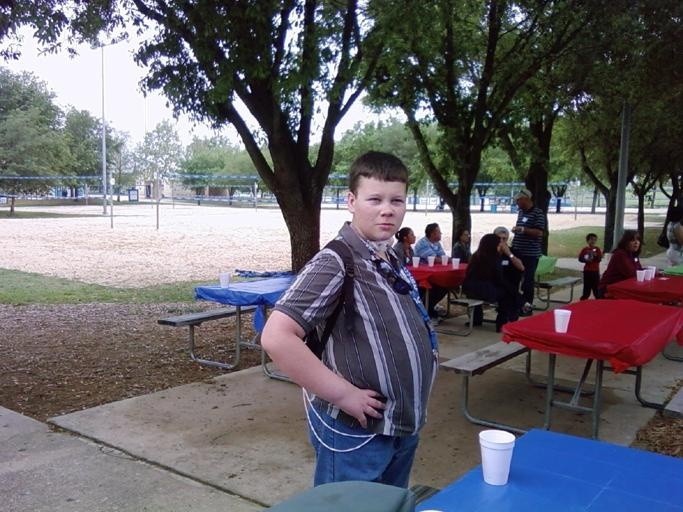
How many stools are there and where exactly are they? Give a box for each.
[266,480,415,512]
[638,388,683,421]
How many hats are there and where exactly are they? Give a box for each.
[513,189,533,200]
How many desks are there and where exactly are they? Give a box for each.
[533,256,557,275]
[194,275,294,380]
[607,266,683,345]
[407,262,470,334]
[416,428,683,512]
[501,299,683,441]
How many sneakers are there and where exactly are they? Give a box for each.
[431,318,439,326]
[521,304,533,314]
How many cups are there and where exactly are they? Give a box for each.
[636,266,656,281]
[219,271,230,288]
[479,429,516,486]
[554,309,572,333]
[412,255,460,269]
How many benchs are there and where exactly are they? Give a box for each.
[437,339,592,434]
[531,276,582,310]
[157,304,275,370]
[435,299,484,336]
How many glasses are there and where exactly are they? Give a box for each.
[373,260,413,295]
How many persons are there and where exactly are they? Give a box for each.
[414,222,451,319]
[510,189,546,306]
[666,206,682,266]
[453,229,472,263]
[393,228,417,266]
[494,227,525,321]
[260,152,439,488]
[463,233,517,332]
[579,233,601,300]
[598,230,647,296]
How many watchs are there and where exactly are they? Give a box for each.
[510,254,514,259]
